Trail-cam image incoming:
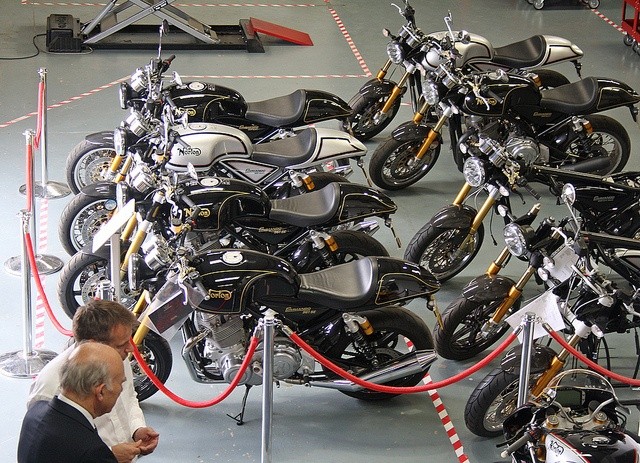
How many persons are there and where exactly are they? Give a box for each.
[27,300,160,462]
[17,340,127,463]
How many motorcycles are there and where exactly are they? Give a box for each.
[404,85,640,285]
[124,189,440,426]
[56,104,402,322]
[66,18,354,198]
[369,9,640,190]
[465,181,639,437]
[493,368,639,463]
[347,0,584,141]
[50,71,373,259]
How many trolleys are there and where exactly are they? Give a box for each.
[526,0,601,8]
[621,0,640,54]
